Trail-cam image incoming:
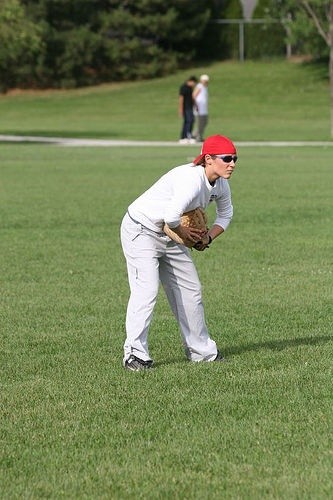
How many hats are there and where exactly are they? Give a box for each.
[193,134,236,165]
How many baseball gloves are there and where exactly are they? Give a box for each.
[162,207,210,252]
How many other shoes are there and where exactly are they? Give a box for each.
[124,355,156,372]
[214,350,226,362]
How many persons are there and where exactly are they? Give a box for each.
[118,134,238,372]
[179,74,208,141]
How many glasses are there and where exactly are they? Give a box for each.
[210,155,238,164]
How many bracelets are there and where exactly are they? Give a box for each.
[208,234,212,244]
